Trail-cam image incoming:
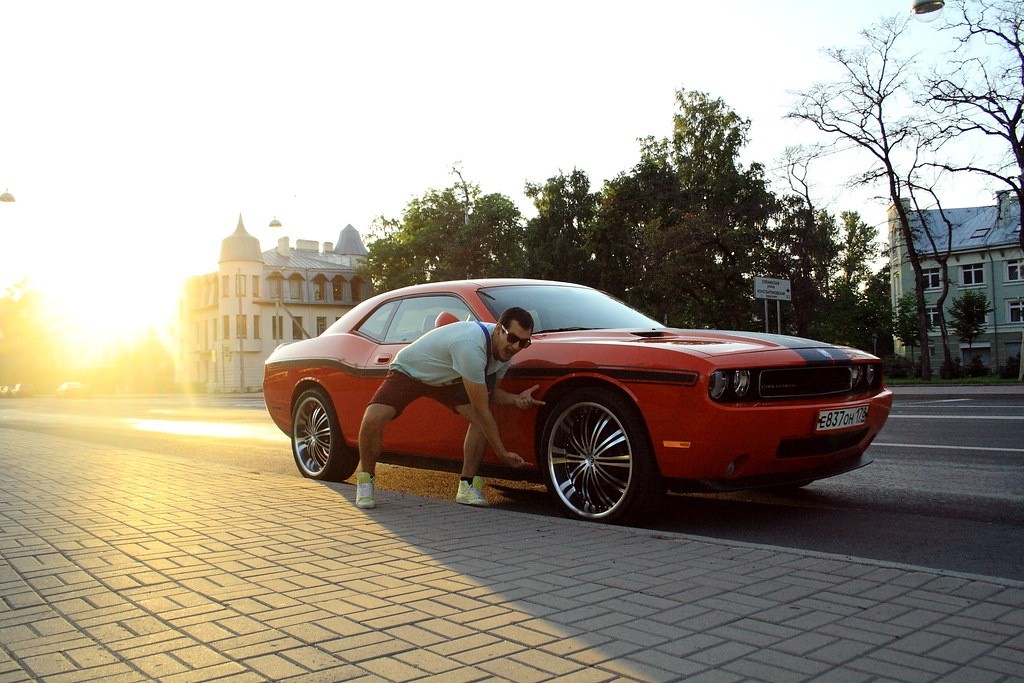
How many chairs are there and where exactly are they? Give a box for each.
[423,315,437,333]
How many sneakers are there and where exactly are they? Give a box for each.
[455,476,488,507]
[356,472,375,509]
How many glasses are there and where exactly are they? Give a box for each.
[502,325,532,348]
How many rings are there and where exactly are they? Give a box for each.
[526,403,528,406]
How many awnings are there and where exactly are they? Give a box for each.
[959,342,991,348]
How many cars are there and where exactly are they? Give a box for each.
[261,277,893,525]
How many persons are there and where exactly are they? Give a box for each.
[356,307,545,509]
